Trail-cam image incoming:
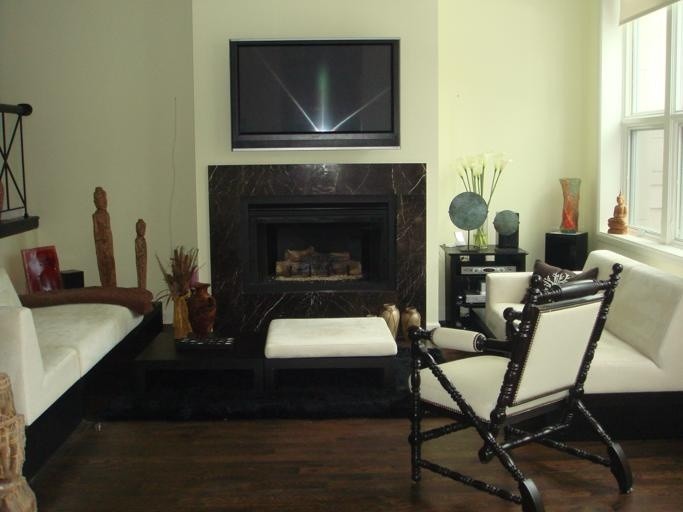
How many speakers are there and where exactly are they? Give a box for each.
[495,213,520,248]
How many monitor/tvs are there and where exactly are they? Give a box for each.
[229,38,401,149]
[60,270,85,290]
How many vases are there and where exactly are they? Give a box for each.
[473,210,488,250]
[558,177,583,234]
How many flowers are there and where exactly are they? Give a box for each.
[456,149,514,209]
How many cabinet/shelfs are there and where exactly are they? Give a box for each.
[443,246,529,327]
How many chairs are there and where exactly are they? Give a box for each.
[408,263,635,512]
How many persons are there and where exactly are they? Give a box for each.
[612,191,627,219]
[90,186,117,287]
[133,217,148,289]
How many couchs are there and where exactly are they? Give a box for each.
[0,264,163,466]
[485,248,682,411]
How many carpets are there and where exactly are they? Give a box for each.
[82,347,456,419]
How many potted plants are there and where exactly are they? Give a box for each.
[161,243,199,340]
